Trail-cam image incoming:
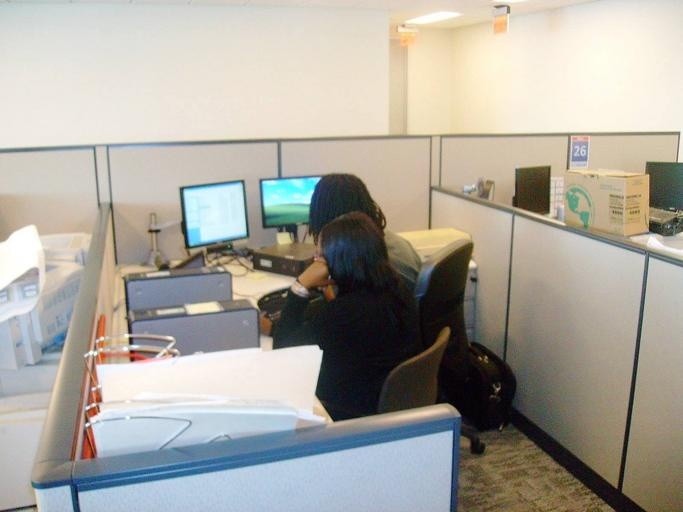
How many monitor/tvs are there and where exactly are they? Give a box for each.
[512,165,551,215]
[180,180,250,249]
[260,176,323,228]
[645,162,683,212]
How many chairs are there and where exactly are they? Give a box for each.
[379,239,495,456]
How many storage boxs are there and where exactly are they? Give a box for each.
[565,168,651,236]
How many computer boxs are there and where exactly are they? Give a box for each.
[648,207,683,236]
[253,241,316,278]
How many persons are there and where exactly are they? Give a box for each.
[306,170,421,294]
[269,209,426,427]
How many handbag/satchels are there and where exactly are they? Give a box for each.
[467,341,516,433]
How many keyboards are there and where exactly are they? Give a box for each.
[257,287,325,321]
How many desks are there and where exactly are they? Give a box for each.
[112,229,469,388]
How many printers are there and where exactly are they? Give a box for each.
[383,226,474,273]
